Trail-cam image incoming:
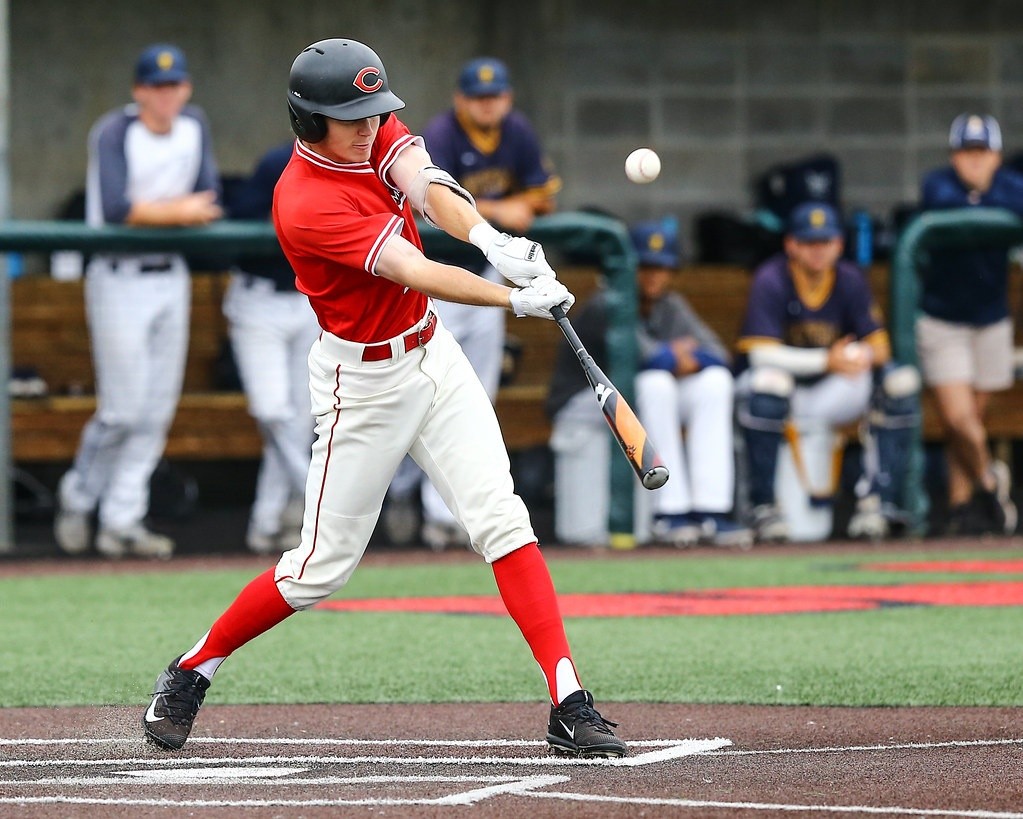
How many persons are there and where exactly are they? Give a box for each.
[541,212,758,547]
[140,38,629,760]
[384,59,563,554]
[731,202,892,546]
[906,108,1018,537]
[216,144,324,556]
[52,46,224,560]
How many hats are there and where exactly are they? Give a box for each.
[946,111,1003,152]
[458,58,510,96]
[135,43,189,85]
[637,232,675,266]
[792,205,842,241]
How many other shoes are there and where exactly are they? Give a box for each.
[652,512,753,546]
[422,522,470,550]
[387,498,413,544]
[980,460,1017,535]
[246,526,301,554]
[848,494,888,540]
[743,506,786,540]
[920,503,968,543]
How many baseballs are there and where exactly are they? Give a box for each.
[624,148,662,184]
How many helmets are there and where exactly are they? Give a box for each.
[286,39,406,144]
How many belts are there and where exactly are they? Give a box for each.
[246,275,298,292]
[321,298,438,362]
[112,262,171,272]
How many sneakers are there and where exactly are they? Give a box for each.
[141,653,211,750]
[97,523,172,560]
[57,510,88,554]
[543,689,627,758]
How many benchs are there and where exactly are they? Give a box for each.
[11,260,1023,542]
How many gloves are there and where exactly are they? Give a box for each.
[486,233,556,287]
[510,275,575,321]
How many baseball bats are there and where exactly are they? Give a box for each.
[550,305,670,490]
